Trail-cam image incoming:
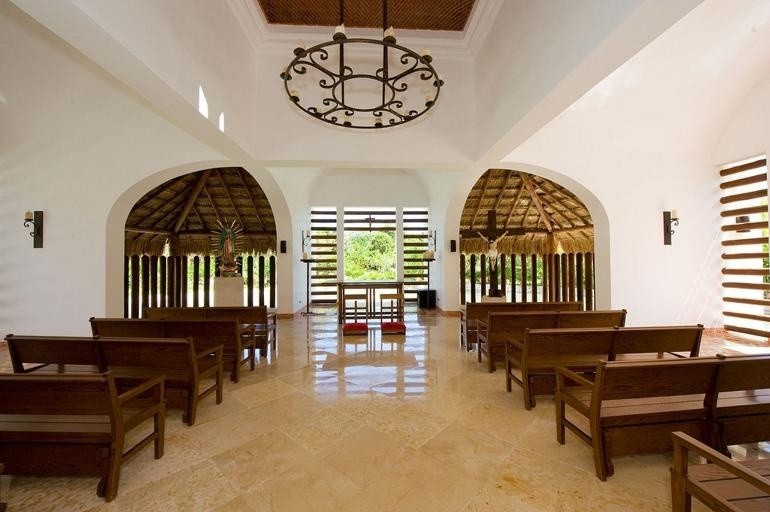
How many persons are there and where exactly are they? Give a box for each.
[476,230,509,272]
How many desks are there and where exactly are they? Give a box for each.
[336,281,405,323]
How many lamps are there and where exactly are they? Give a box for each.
[300,252,315,317]
[280,1,445,131]
[422,249,436,310]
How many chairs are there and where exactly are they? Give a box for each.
[379,293,406,336]
[342,293,369,336]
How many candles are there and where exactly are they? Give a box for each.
[23,209,34,220]
[670,209,678,219]
[428,229,433,236]
[306,229,311,236]
